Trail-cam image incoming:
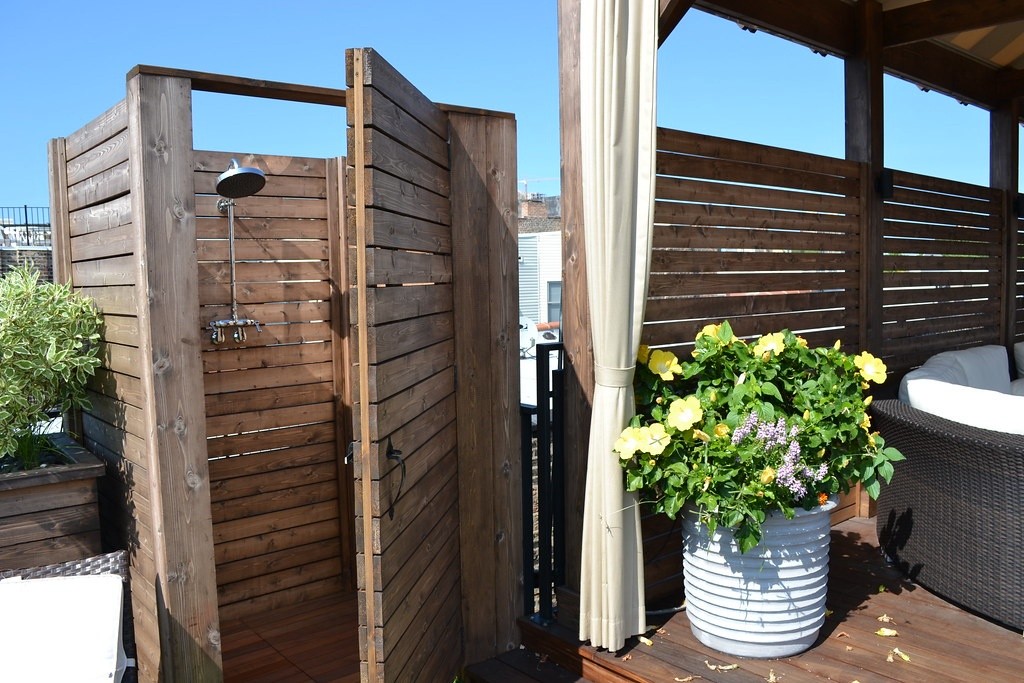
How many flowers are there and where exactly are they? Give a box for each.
[607,296,899,555]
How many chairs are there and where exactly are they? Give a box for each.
[0,550,139,683]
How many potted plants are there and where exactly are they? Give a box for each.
[0,256,108,573]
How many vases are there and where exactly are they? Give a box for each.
[675,488,841,660]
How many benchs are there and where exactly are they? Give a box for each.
[869,334,1024,639]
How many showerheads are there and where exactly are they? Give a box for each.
[215,167,267,199]
